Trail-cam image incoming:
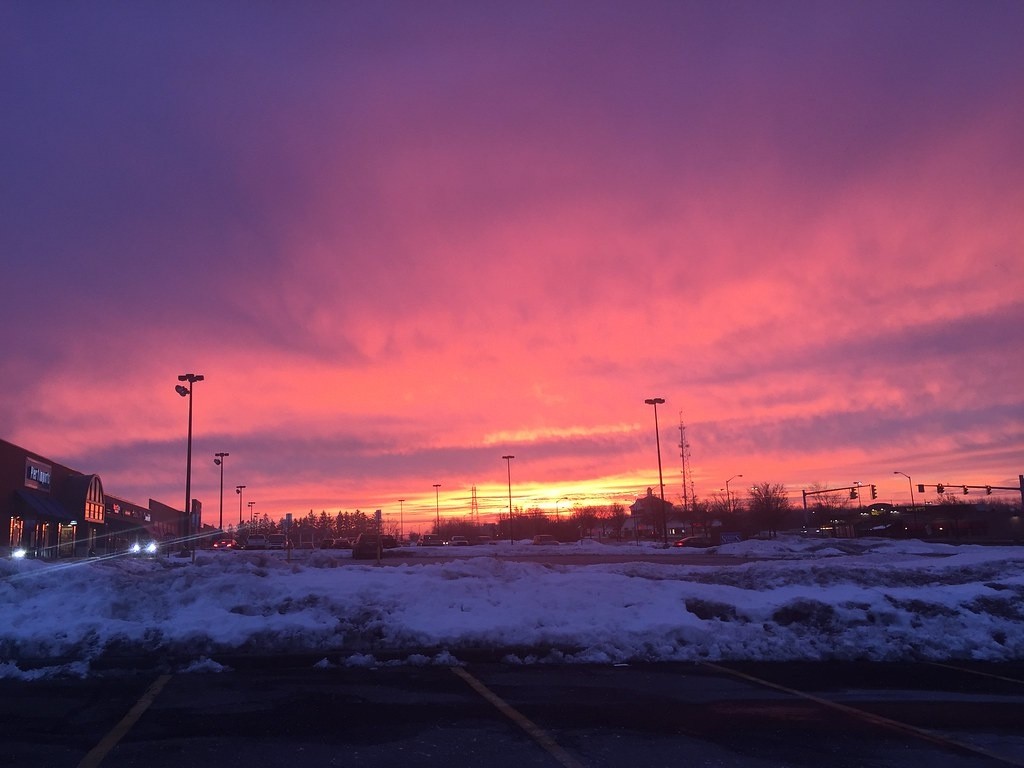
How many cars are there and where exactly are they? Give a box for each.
[267,534,295,550]
[130,539,162,557]
[533,535,560,544]
[672,536,719,547]
[416,534,443,546]
[469,535,497,545]
[322,534,398,548]
[245,534,267,548]
[446,536,467,546]
[213,539,240,549]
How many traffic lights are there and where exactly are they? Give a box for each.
[850,488,858,500]
[963,485,969,495]
[986,486,992,495]
[871,484,877,499]
[938,485,944,493]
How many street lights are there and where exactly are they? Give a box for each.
[894,472,914,511]
[214,453,229,531]
[556,496,568,534]
[236,485,246,526]
[649,484,666,542]
[398,500,405,541]
[256,513,260,522]
[645,398,670,549]
[726,474,743,512]
[178,373,203,558]
[249,502,255,522]
[720,488,736,512]
[433,484,442,535]
[854,481,862,508]
[502,455,515,544]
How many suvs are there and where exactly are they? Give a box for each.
[352,532,384,559]
